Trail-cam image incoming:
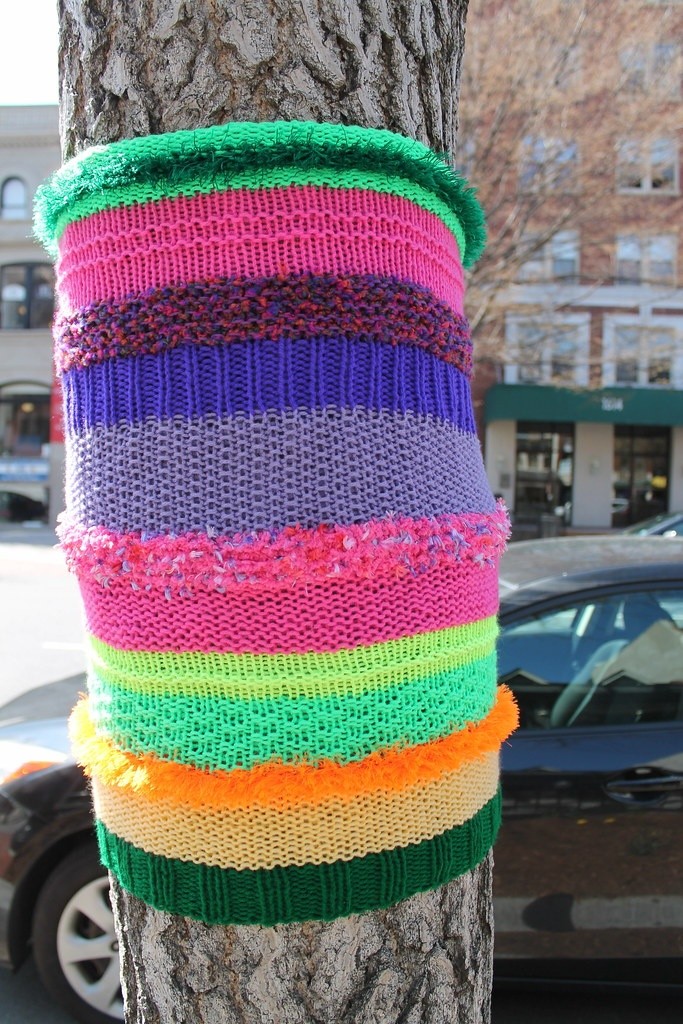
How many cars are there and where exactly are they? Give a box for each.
[0,510,683,1024]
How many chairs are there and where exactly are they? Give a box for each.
[550,601,674,728]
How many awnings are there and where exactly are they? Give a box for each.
[483,382,683,434]
